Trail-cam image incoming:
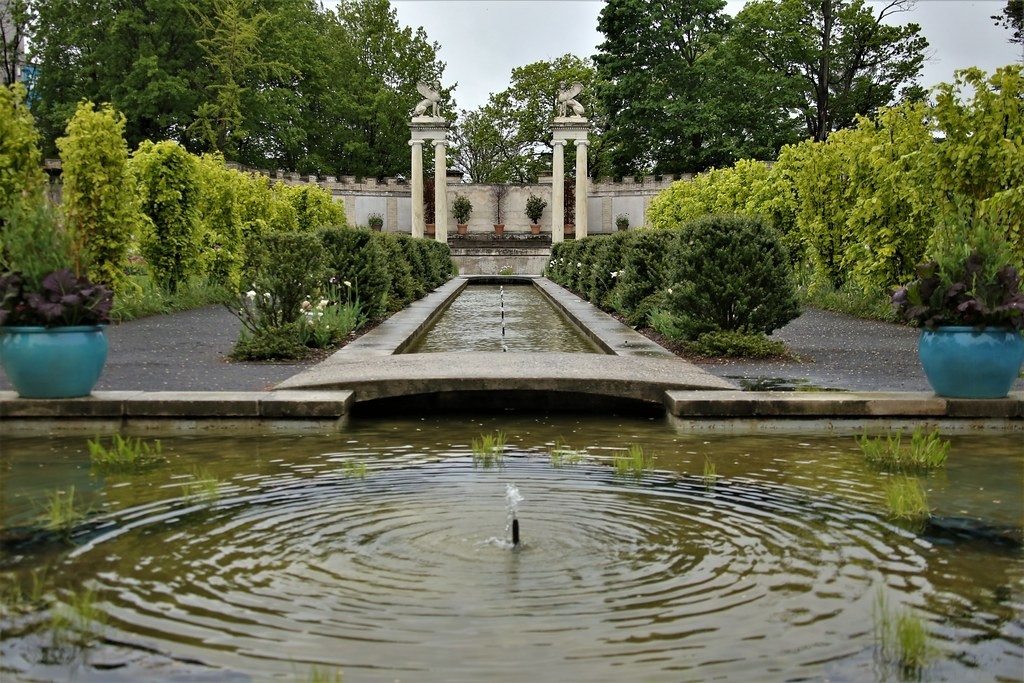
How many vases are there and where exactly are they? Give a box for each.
[493,224,505,236]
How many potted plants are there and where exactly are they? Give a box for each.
[0,272,115,398]
[423,178,435,234]
[888,251,1024,399]
[563,174,577,234]
[450,195,473,235]
[616,213,630,232]
[524,192,547,234]
[368,213,384,233]
